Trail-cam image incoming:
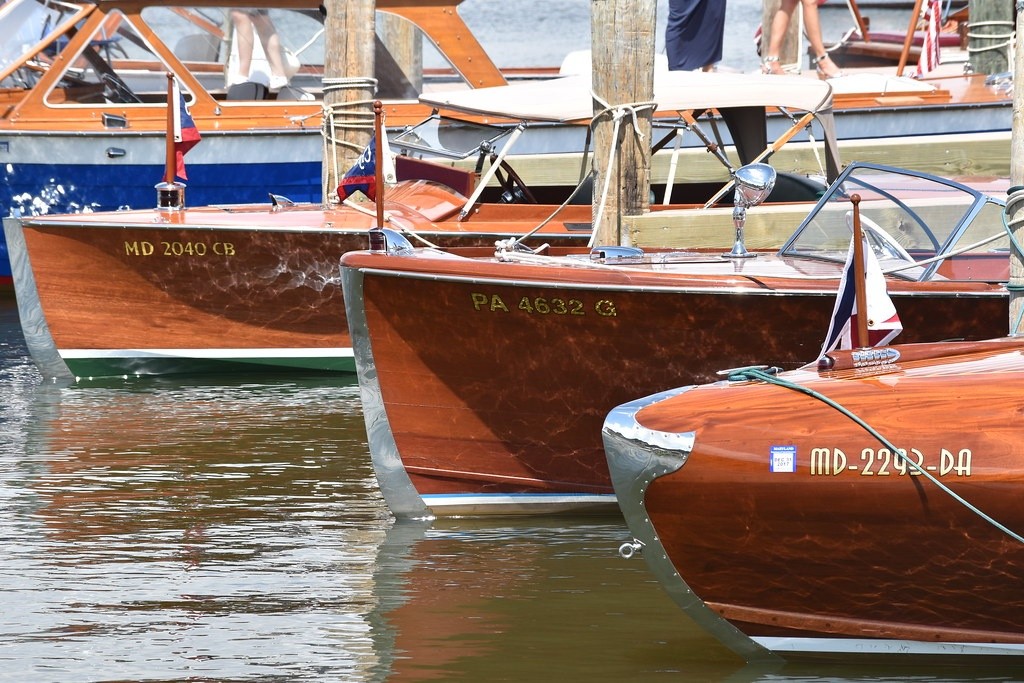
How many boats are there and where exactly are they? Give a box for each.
[0,1,1024,658]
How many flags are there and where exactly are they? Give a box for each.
[815,223,903,360]
[162,76,201,184]
[915,0,942,75]
[330,117,397,204]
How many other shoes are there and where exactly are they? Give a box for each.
[269,83,315,100]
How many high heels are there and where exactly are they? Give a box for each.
[761,57,784,75]
[814,52,848,80]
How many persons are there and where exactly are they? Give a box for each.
[224,9,292,90]
[762,0,848,81]
[665,0,727,72]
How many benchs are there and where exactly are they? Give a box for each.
[388,108,513,194]
